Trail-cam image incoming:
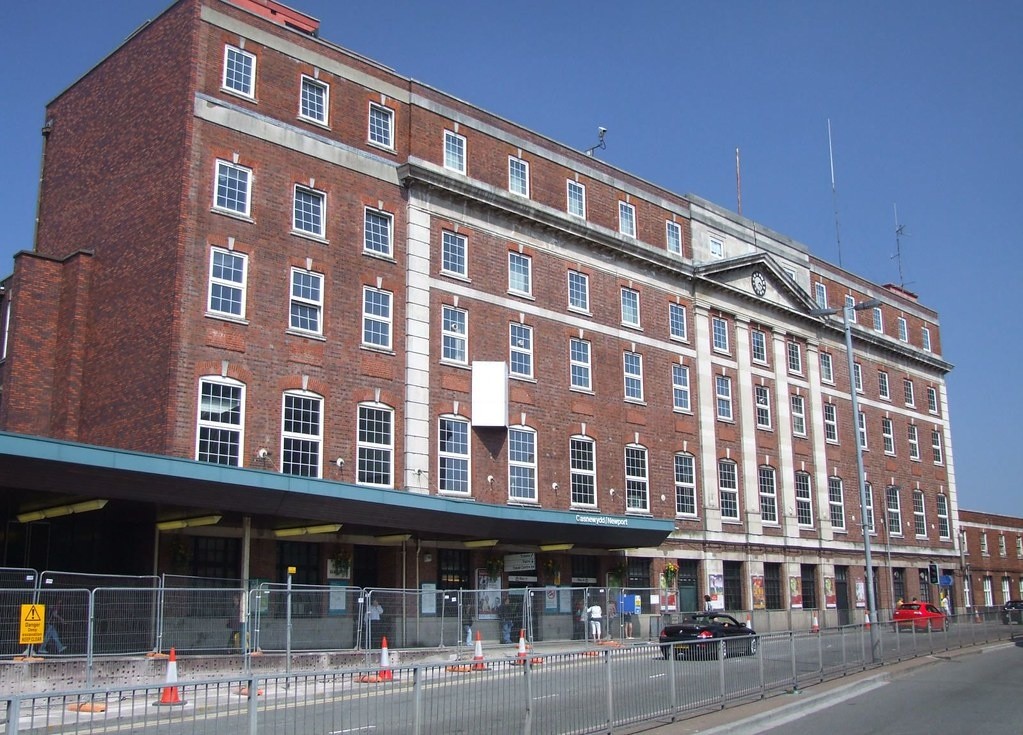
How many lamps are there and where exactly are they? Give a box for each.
[522,542,537,552]
[274,527,307,538]
[462,539,499,548]
[71,499,108,513]
[16,510,46,523]
[374,534,414,543]
[186,515,223,527]
[305,523,343,535]
[43,506,73,519]
[157,520,188,531]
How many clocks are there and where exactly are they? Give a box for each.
[751,270,766,297]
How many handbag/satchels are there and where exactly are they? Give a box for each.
[575,609,581,617]
[227,620,232,628]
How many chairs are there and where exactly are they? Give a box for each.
[683,620,701,624]
[703,621,725,628]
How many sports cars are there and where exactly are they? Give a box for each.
[659,613,759,660]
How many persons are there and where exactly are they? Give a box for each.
[498,596,514,644]
[624,612,632,638]
[38,593,67,654]
[226,594,249,654]
[896,594,952,623]
[364,597,384,648]
[704,595,713,611]
[577,599,616,642]
[462,603,474,646]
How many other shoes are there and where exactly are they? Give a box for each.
[227,652,233,655]
[467,642,474,646]
[504,641,512,645]
[59,646,66,652]
[626,636,633,640]
[38,650,48,653]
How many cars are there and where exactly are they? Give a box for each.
[893,602,949,633]
[1001,600,1023,625]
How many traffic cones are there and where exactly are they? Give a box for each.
[378,637,399,682]
[812,611,819,633]
[510,629,532,666]
[974,606,982,623]
[864,610,871,631]
[151,647,187,706]
[746,614,752,629]
[472,630,492,671]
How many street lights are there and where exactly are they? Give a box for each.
[809,299,882,664]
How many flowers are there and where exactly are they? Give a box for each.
[541,559,562,577]
[663,560,681,583]
[165,534,195,572]
[485,558,504,583]
[331,547,354,574]
